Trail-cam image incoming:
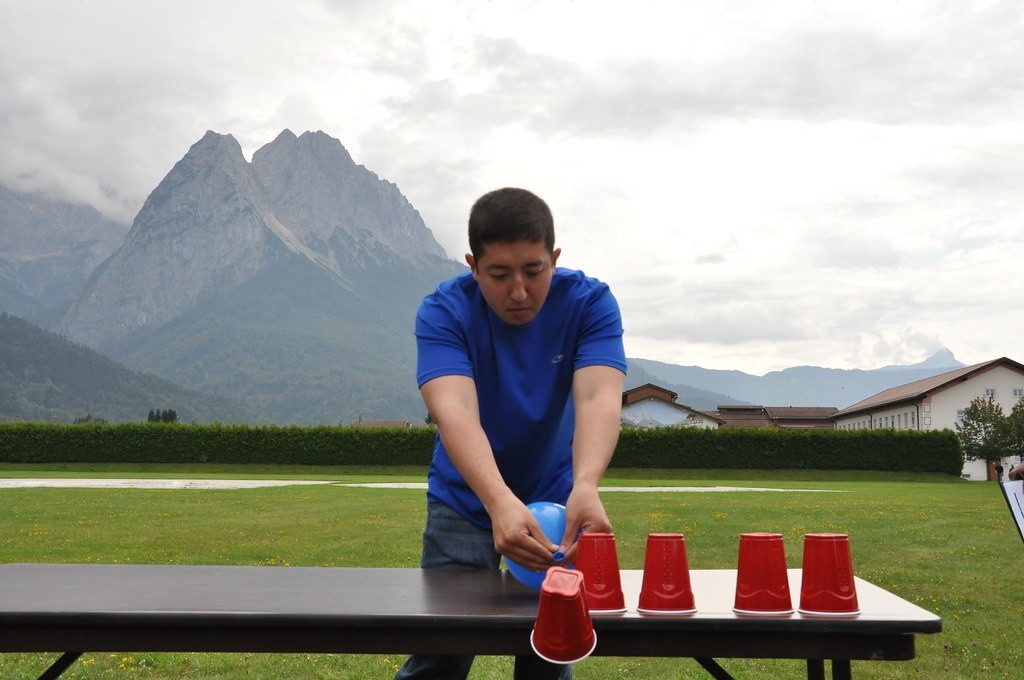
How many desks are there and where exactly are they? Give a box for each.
[0,564,942,680]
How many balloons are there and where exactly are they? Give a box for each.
[504,501,585,592]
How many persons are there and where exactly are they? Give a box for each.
[392,189,628,680]
[1009,464,1015,472]
[995,462,1004,485]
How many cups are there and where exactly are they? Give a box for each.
[798,533,861,616]
[575,533,628,616]
[732,533,792,617]
[531,567,597,665]
[636,534,697,616]
